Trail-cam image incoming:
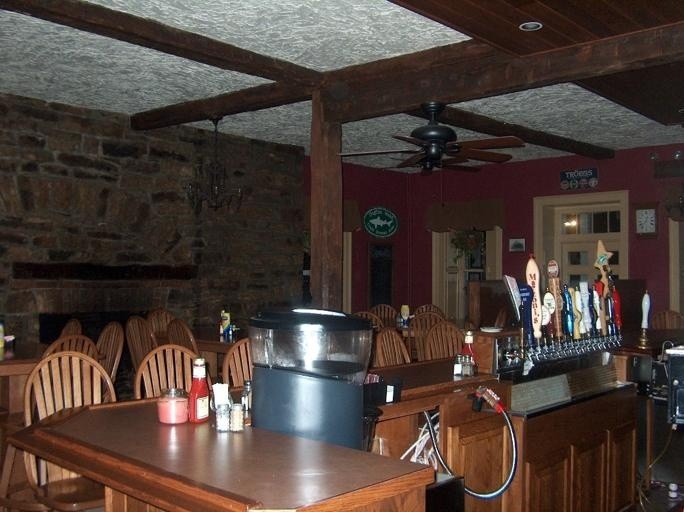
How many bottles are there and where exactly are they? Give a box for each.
[187,356,210,424]
[396,313,403,329]
[0,320,5,348]
[230,405,244,431]
[240,381,253,426]
[218,310,240,336]
[0,349,5,360]
[400,304,410,329]
[214,403,230,432]
[451,334,625,377]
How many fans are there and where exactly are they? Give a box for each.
[336,102,526,180]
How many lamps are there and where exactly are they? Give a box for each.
[187,114,243,216]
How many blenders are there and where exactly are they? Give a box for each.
[246,307,374,450]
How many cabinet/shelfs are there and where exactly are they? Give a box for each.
[444,383,503,512]
[503,385,638,512]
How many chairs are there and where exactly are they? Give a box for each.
[147,307,177,345]
[23,354,117,511]
[126,313,157,370]
[95,318,128,382]
[135,343,214,400]
[221,300,466,394]
[42,333,99,363]
[61,318,84,339]
[166,316,201,362]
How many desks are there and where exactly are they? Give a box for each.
[7,328,684,512]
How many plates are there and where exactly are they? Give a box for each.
[479,326,503,333]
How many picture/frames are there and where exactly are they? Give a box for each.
[508,237,526,253]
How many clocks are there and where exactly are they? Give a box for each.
[633,201,659,240]
